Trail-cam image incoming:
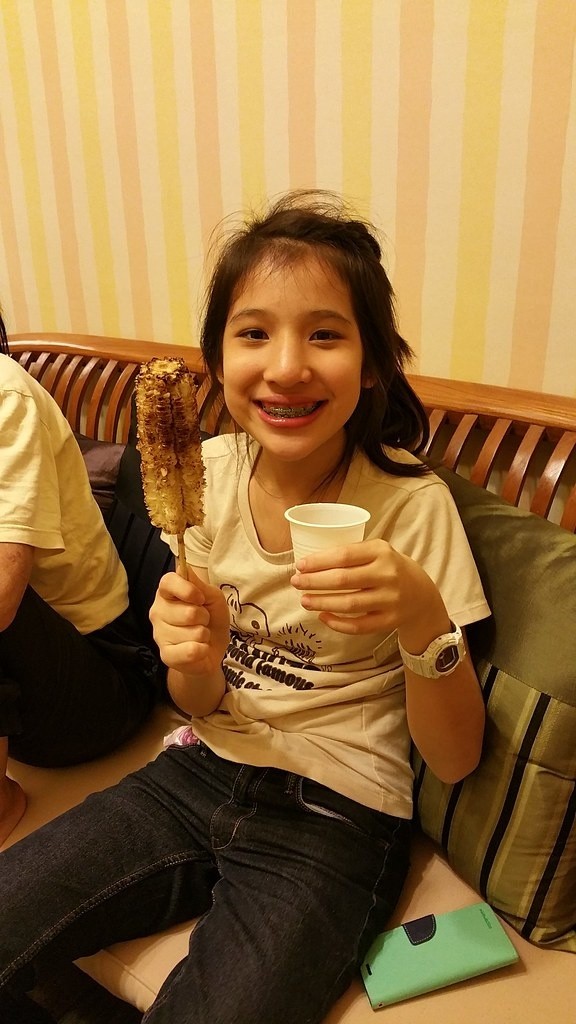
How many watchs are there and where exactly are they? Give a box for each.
[373,616,468,680]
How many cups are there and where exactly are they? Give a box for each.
[284,503,370,613]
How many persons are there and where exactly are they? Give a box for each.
[0,318,155,847]
[0,190,491,1024]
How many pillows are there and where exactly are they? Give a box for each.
[410,452,576,956]
[70,392,214,724]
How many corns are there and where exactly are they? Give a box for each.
[135,356,208,534]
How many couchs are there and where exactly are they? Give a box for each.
[1,328,574,1024]
